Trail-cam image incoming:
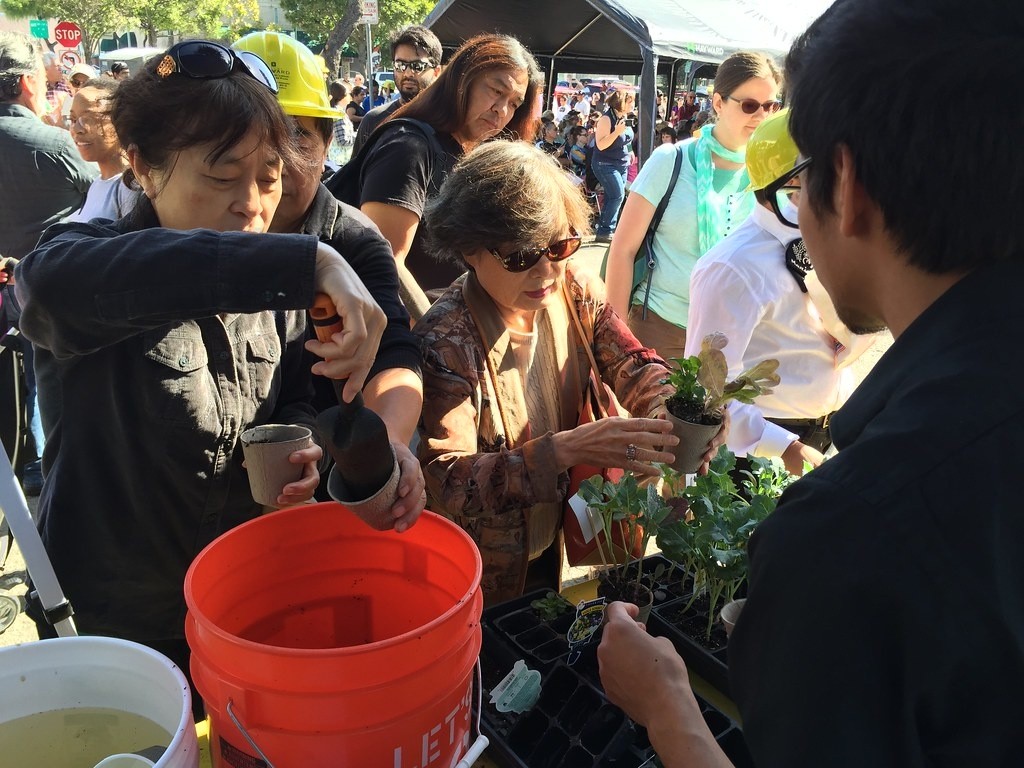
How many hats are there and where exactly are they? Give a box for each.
[68,64,99,79]
[707,84,714,93]
[314,52,330,73]
[574,90,585,95]
[567,109,580,115]
[381,79,397,90]
[656,90,663,97]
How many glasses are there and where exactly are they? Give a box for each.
[548,127,557,132]
[148,39,279,95]
[70,79,84,87]
[60,114,115,130]
[762,155,818,229]
[579,133,586,138]
[726,94,782,115]
[390,59,436,75]
[485,224,582,274]
[687,96,696,100]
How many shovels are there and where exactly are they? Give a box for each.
[308,292,394,501]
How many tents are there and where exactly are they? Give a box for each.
[420,1,835,174]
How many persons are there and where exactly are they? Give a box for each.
[356,31,539,455]
[412,137,730,600]
[0,43,141,495]
[536,74,712,243]
[683,106,880,477]
[351,26,442,161]
[316,54,395,167]
[229,33,427,532]
[605,51,835,522]
[596,1,1024,768]
[16,38,387,725]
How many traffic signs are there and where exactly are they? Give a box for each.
[359,0,379,25]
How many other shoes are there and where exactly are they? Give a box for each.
[22,461,45,496]
[594,233,613,243]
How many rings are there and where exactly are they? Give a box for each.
[625,444,638,460]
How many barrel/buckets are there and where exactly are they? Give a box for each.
[0,637,200,768]
[183,501,490,768]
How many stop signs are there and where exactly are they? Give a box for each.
[55,22,82,47]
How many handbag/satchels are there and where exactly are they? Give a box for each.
[598,143,683,306]
[328,135,353,166]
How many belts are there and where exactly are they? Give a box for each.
[767,412,837,428]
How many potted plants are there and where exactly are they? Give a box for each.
[657,331,781,475]
[576,444,673,629]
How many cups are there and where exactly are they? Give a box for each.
[327,441,401,531]
[94,746,167,768]
[240,424,312,506]
[721,598,747,638]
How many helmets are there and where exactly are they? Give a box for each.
[739,107,799,192]
[229,30,346,122]
